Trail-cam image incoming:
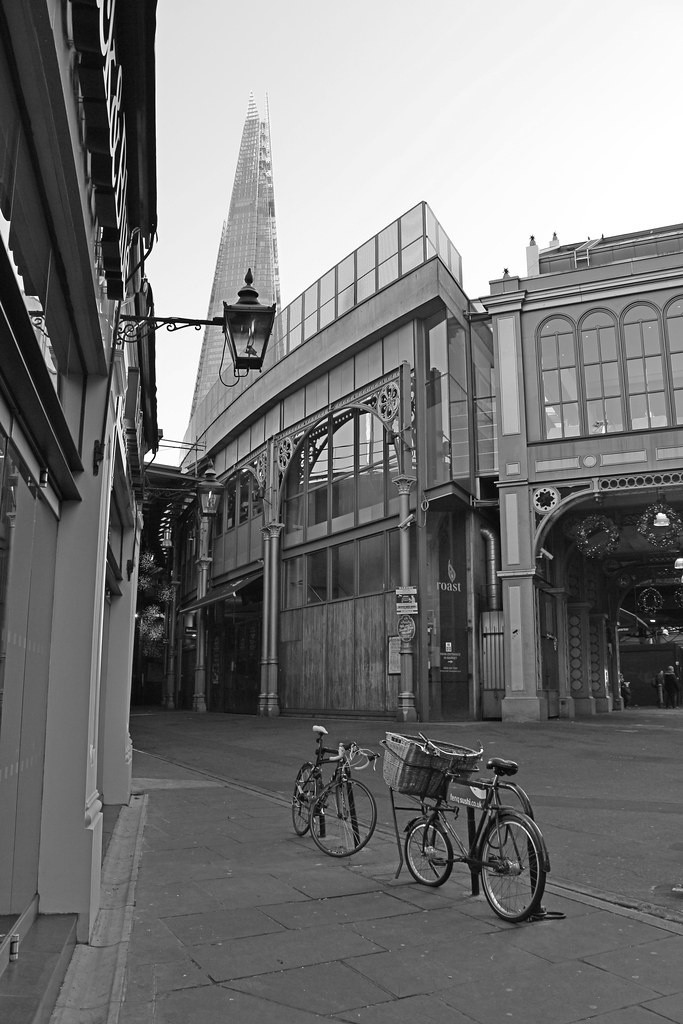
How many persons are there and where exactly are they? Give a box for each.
[663,665,680,710]
[620,677,631,710]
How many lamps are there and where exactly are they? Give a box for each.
[653,487,670,526]
[39,467,48,489]
[673,557,683,570]
[129,457,223,517]
[119,265,277,387]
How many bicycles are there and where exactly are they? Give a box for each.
[290,725,381,857]
[378,731,551,924]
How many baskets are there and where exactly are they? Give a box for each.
[383,732,480,797]
